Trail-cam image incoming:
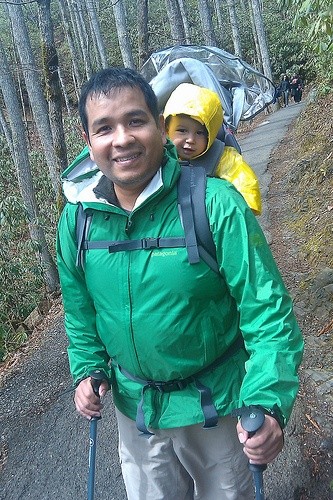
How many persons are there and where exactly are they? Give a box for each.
[53,66,306,499]
[157,83,264,215]
[289,72,304,104]
[278,75,291,108]
